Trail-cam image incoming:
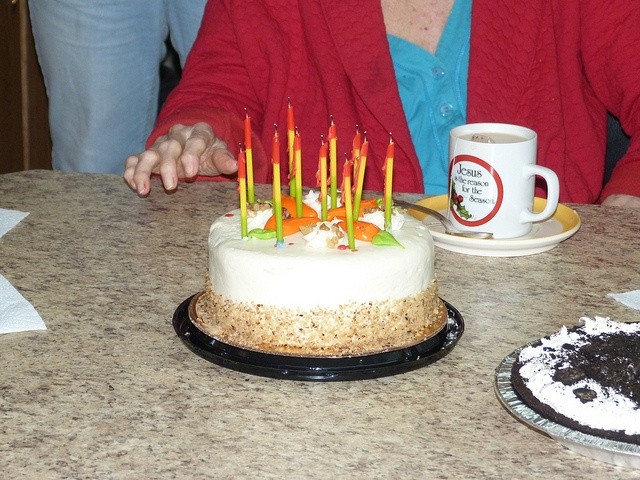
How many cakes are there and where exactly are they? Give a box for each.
[511,315,640,446]
[188,97,448,360]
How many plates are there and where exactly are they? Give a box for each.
[401,190,580,256]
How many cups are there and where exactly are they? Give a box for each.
[448,123,560,237]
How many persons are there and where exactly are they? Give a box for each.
[26,0,206,172]
[124,0,640,207]
